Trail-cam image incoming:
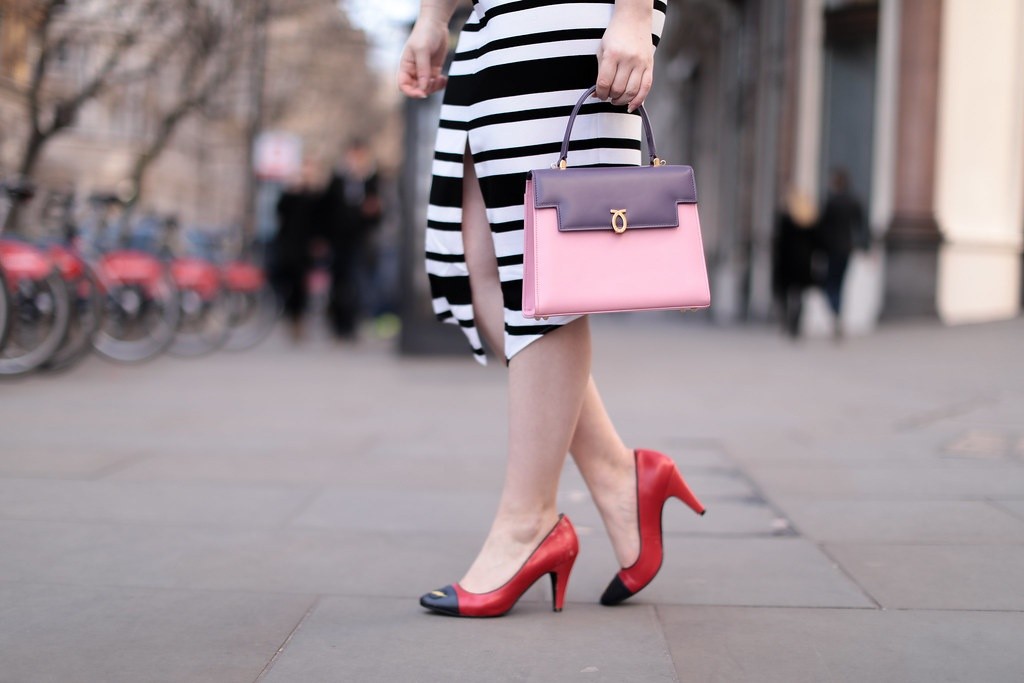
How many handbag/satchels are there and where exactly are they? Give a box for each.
[522,81,713,317]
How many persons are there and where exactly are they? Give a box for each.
[263,140,389,341]
[397,0,708,618]
[768,187,813,337]
[813,166,867,336]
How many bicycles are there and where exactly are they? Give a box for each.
[0,178,288,381]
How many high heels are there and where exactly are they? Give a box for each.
[421,512,578,617]
[599,448,706,606]
